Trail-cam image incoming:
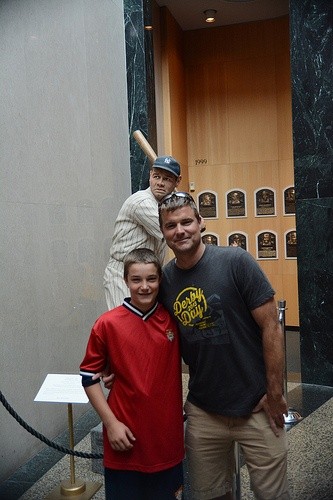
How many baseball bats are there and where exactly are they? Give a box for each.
[133,130,158,164]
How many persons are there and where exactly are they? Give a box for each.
[92,191,289,500]
[103,155,182,311]
[79,248,185,500]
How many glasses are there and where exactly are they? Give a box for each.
[158,192,195,209]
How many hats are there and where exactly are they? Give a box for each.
[152,156,180,178]
